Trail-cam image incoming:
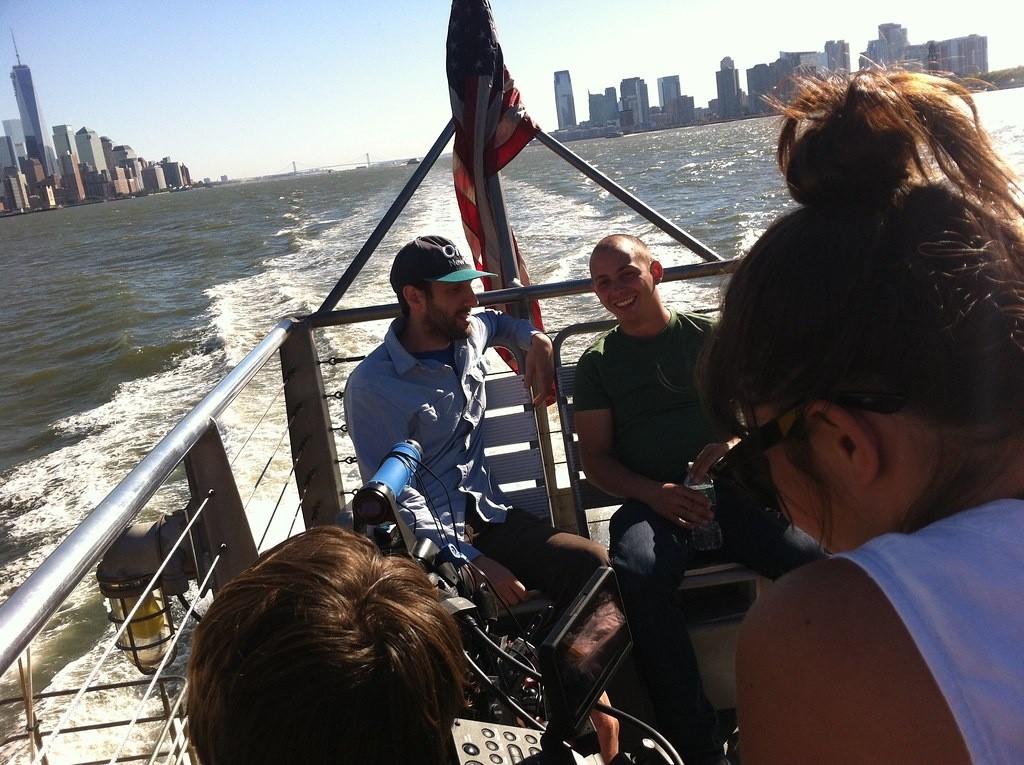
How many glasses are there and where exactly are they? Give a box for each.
[705,392,906,499]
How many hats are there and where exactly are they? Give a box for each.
[390,235,498,294]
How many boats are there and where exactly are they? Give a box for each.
[605,131,624,139]
[407,158,419,164]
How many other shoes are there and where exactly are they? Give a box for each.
[680,703,732,765]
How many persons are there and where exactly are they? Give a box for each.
[694,73,1024,765]
[344,236,611,622]
[188,525,619,765]
[574,236,825,765]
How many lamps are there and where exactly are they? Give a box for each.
[96,508,197,676]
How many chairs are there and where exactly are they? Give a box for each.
[553,308,770,599]
[473,339,559,615]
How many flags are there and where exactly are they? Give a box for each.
[446,1,546,376]
